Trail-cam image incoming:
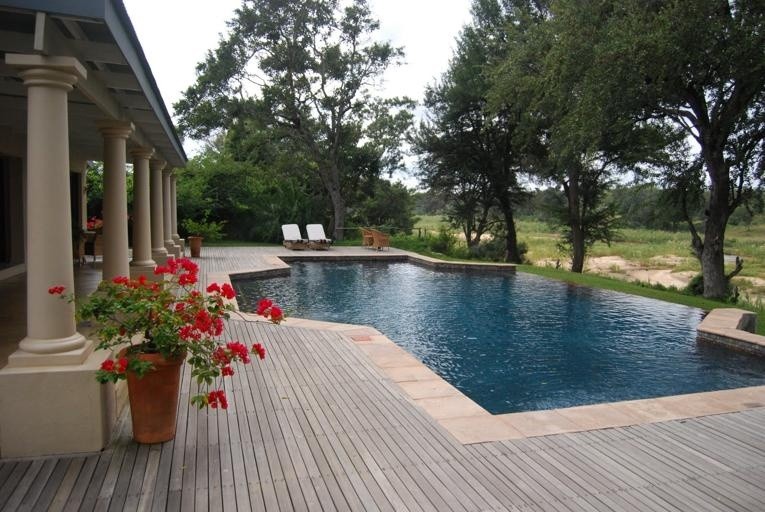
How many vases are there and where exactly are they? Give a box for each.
[116,341,187,446]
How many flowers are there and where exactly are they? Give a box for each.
[45,252,289,413]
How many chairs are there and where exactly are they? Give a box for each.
[359,225,378,248]
[89,228,106,268]
[70,229,88,267]
[370,229,391,252]
[306,222,333,251]
[280,223,308,251]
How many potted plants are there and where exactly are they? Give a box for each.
[180,207,229,258]
